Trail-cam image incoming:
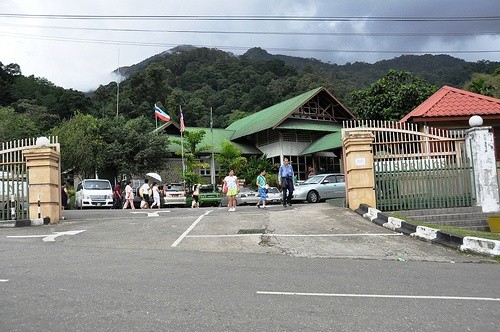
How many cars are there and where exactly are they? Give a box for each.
[281,173,346,203]
[235,186,282,206]
[159,183,187,208]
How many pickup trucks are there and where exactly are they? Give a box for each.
[186,184,223,208]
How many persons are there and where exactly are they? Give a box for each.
[222,169,240,212]
[278,158,294,207]
[120,181,136,210]
[150,182,169,209]
[307,165,326,178]
[140,179,153,210]
[191,183,203,208]
[61,182,70,210]
[294,174,299,187]
[110,183,123,209]
[255,169,269,211]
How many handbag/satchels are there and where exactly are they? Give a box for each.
[122,190,126,195]
[266,189,269,194]
[192,201,196,206]
[223,186,228,194]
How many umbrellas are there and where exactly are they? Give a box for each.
[146,171,163,185]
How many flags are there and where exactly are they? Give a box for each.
[154,106,170,121]
[180,110,185,132]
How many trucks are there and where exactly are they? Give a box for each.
[119,179,154,209]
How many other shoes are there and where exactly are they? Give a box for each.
[232,207,236,211]
[288,203,292,206]
[256,204,260,208]
[191,206,193,208]
[150,206,152,209]
[263,207,268,210]
[228,208,232,211]
[284,204,287,207]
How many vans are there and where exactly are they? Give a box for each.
[0,171,29,220]
[74,179,114,209]
